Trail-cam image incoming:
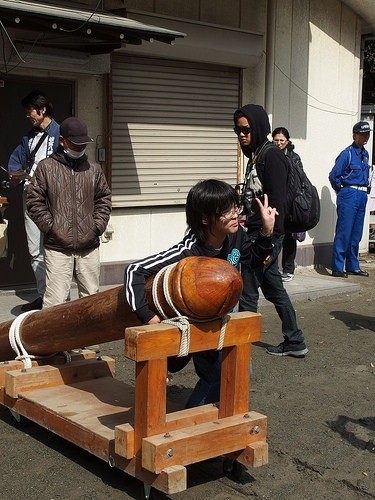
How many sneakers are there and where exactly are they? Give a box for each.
[282,272,294,282]
[265,340,309,355]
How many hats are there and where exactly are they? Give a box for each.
[60,117,96,144]
[353,122,372,132]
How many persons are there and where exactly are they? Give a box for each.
[122,179,281,414]
[270,127,303,282]
[325,121,373,278]
[233,103,309,356]
[27,116,112,312]
[8,94,62,310]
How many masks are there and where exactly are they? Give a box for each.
[63,140,87,159]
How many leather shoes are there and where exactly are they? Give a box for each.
[347,270,369,277]
[21,297,43,311]
[332,270,348,277]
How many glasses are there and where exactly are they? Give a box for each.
[234,126,251,135]
[221,206,244,219]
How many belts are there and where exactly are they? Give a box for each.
[349,185,367,192]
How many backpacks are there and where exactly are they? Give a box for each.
[258,144,320,233]
